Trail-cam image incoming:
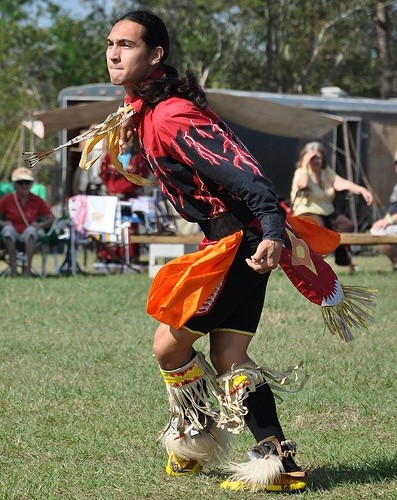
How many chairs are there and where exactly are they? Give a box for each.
[0,182,57,280]
[67,194,131,278]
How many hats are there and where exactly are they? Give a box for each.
[11,166,34,182]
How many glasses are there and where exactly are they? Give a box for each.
[15,180,33,185]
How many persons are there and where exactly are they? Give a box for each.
[370,152,397,272]
[0,167,55,278]
[22,11,306,491]
[290,141,373,274]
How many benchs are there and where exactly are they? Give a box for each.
[129,231,397,281]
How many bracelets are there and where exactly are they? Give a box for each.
[387,215,393,225]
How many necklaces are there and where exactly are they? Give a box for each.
[22,103,136,167]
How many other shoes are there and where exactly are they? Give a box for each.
[165,451,203,477]
[220,465,306,494]
